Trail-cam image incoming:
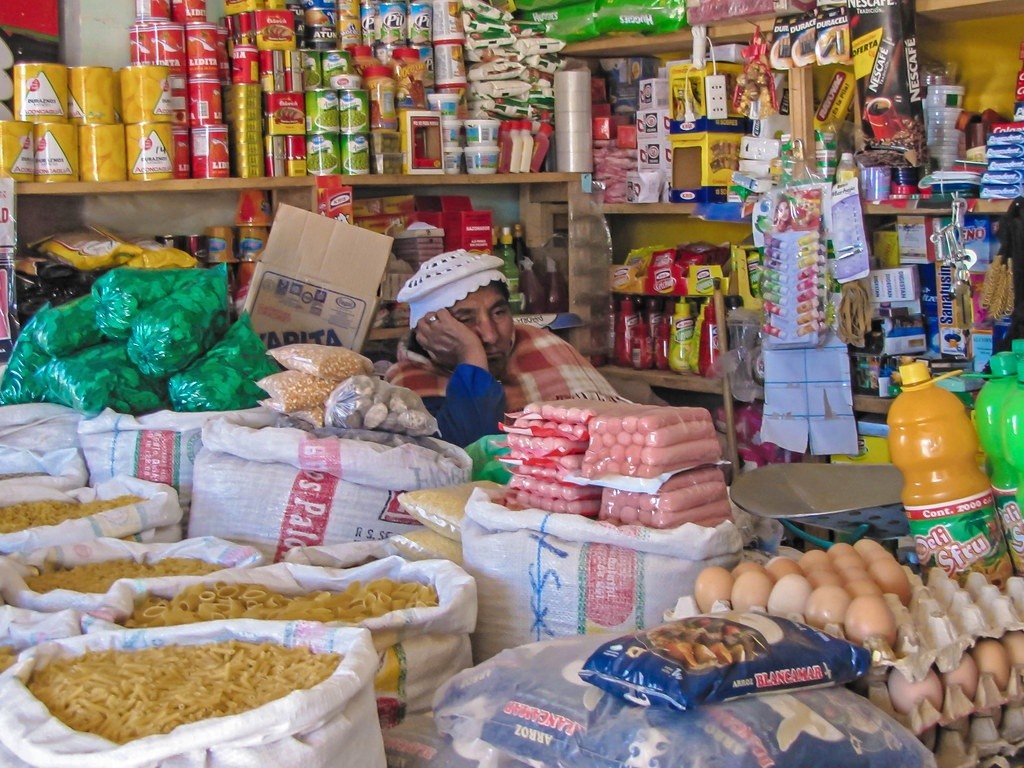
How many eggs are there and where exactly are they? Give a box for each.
[695,539,1024,752]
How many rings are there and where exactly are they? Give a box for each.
[426,316,439,324]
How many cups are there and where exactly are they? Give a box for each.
[866,97,904,141]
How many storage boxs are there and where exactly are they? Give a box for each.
[244,43,1024,407]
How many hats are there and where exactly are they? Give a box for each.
[396,248,510,330]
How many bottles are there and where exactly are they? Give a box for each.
[887,361,1013,594]
[1012,339,1024,360]
[492,224,527,314]
[836,152,860,185]
[1001,361,1024,578]
[976,351,1015,543]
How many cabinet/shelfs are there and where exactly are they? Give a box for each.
[562,0,1024,464]
[14,171,587,342]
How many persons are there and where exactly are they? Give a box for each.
[383,249,617,448]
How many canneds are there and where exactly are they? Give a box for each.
[0,0,474,186]
[156,224,273,305]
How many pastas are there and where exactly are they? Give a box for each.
[121,580,438,621]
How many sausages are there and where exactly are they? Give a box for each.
[503,399,735,530]
